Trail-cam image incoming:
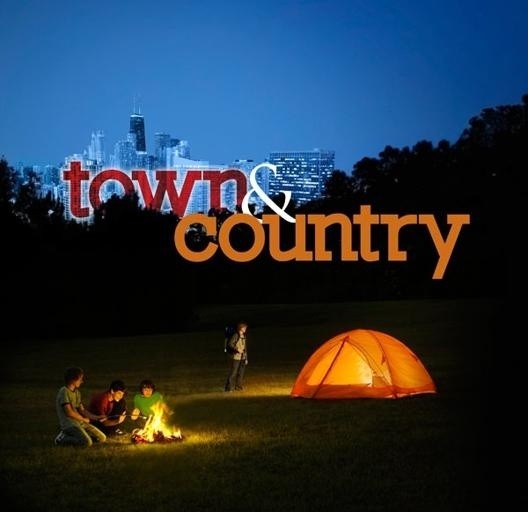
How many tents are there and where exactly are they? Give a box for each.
[290,328,439,402]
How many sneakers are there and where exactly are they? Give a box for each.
[54,431,65,445]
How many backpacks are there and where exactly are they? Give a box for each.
[224,327,240,354]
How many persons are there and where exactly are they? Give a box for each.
[133,380,176,428]
[225,323,249,392]
[83,381,141,436]
[54,366,106,448]
